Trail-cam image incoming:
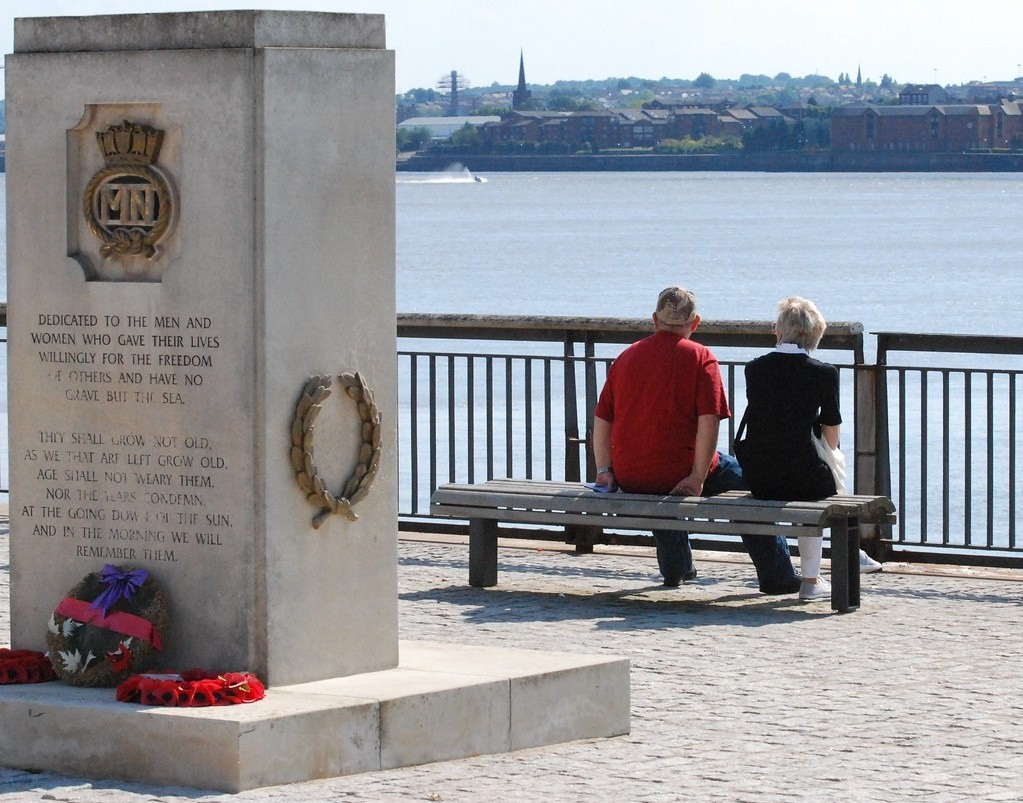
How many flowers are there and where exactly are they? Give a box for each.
[0,648,54,685]
[116,669,266,708]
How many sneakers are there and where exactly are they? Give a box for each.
[799,578,834,599]
[858,549,881,573]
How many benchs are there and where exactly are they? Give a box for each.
[430,478,897,614]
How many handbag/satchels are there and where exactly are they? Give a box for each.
[733,439,746,469]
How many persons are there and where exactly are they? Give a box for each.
[593,286,802,594]
[744,296,881,602]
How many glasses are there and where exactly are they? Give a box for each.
[771,322,777,336]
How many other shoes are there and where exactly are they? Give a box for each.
[663,566,697,587]
[766,568,805,595]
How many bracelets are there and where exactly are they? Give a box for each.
[597,466,613,475]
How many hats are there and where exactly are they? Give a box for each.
[656,286,696,326]
